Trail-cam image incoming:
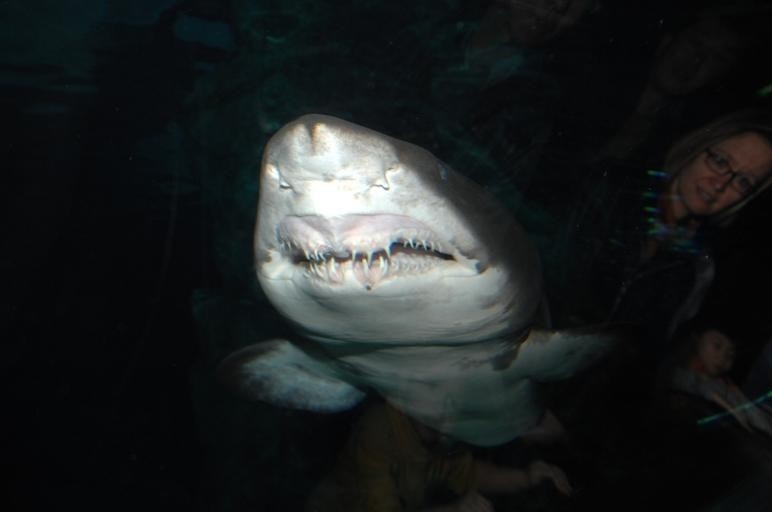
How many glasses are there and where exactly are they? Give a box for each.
[705,146,757,198]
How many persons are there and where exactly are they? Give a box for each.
[302,0,772,512]
